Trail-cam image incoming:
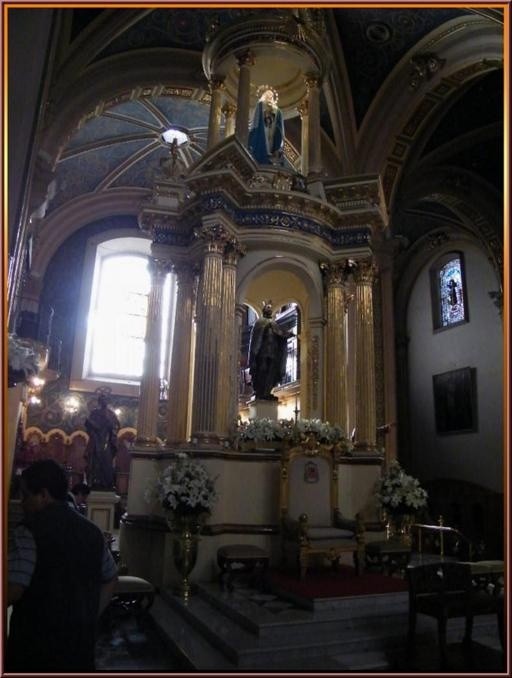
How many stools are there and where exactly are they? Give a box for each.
[214,545,274,592]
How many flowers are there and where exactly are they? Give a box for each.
[375,457,429,511]
[145,452,218,533]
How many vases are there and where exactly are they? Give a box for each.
[381,499,417,547]
[167,509,206,600]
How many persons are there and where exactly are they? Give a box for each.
[83,395,122,492]
[65,483,90,516]
[435,376,472,432]
[248,303,288,401]
[247,89,289,167]
[7,458,120,671]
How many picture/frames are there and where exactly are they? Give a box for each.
[431,366,481,438]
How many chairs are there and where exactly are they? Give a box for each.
[403,560,508,671]
[274,433,368,585]
